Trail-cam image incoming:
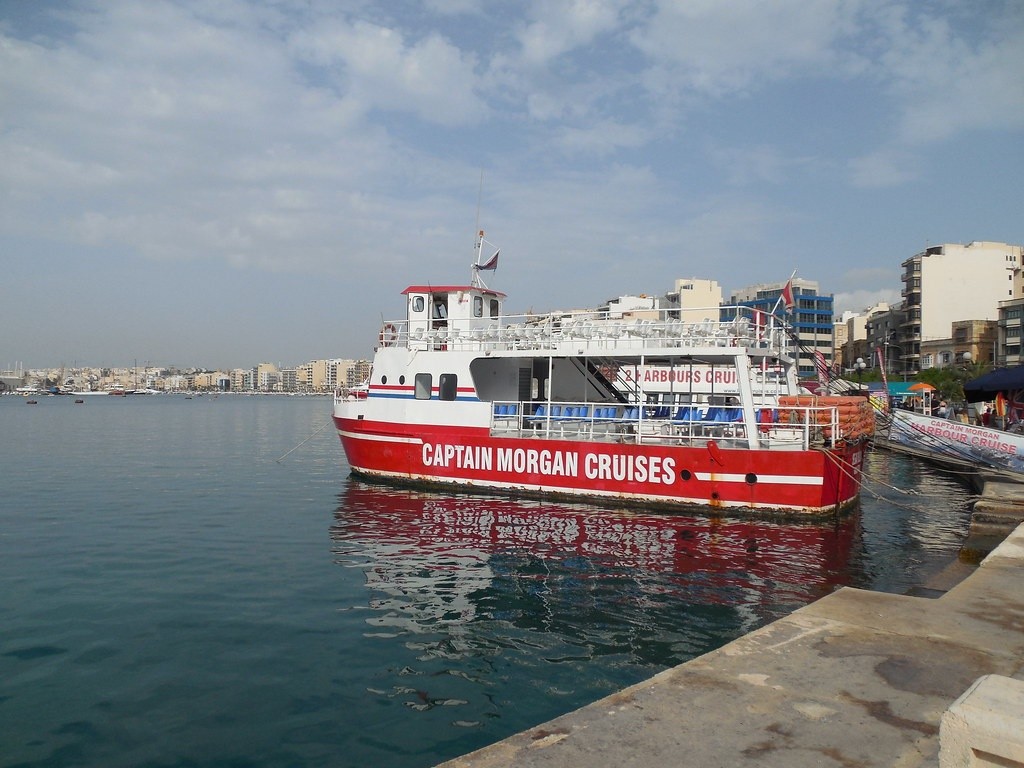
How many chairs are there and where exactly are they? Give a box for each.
[495,404,779,448]
[409,316,779,347]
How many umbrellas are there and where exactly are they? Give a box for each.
[908,383,936,407]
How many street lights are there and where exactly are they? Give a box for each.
[853,357,866,396]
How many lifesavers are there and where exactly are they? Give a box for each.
[378,323,397,347]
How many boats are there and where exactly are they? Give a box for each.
[329,228,878,521]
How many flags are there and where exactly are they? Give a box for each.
[780,279,796,316]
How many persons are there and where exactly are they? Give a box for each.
[961,408,1024,435]
[903,396,948,418]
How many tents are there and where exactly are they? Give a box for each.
[814,378,869,394]
[963,364,1024,404]
